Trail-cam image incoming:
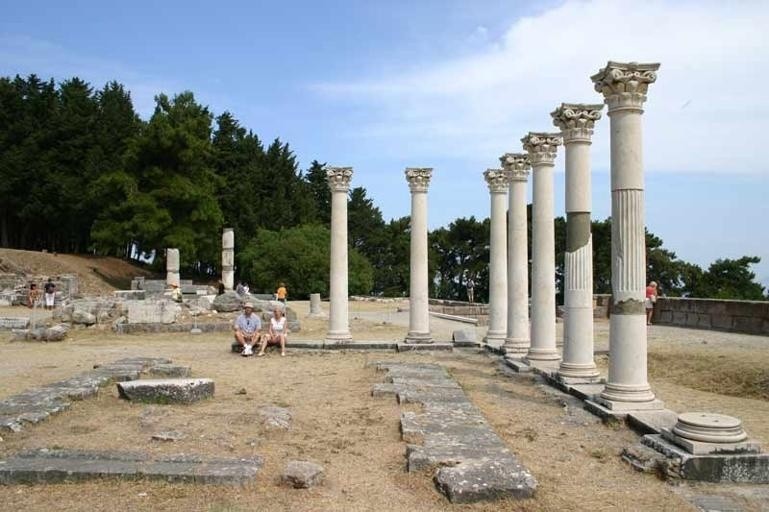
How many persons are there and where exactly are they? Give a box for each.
[258,305,289,357]
[169,282,182,303]
[43,279,56,310]
[233,302,263,357]
[645,280,659,325]
[277,282,288,303]
[27,283,39,310]
[235,279,245,295]
[243,283,250,294]
[218,278,227,297]
[465,276,475,304]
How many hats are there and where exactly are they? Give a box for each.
[243,302,254,309]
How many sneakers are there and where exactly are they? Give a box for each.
[280,351,284,356]
[241,347,265,356]
